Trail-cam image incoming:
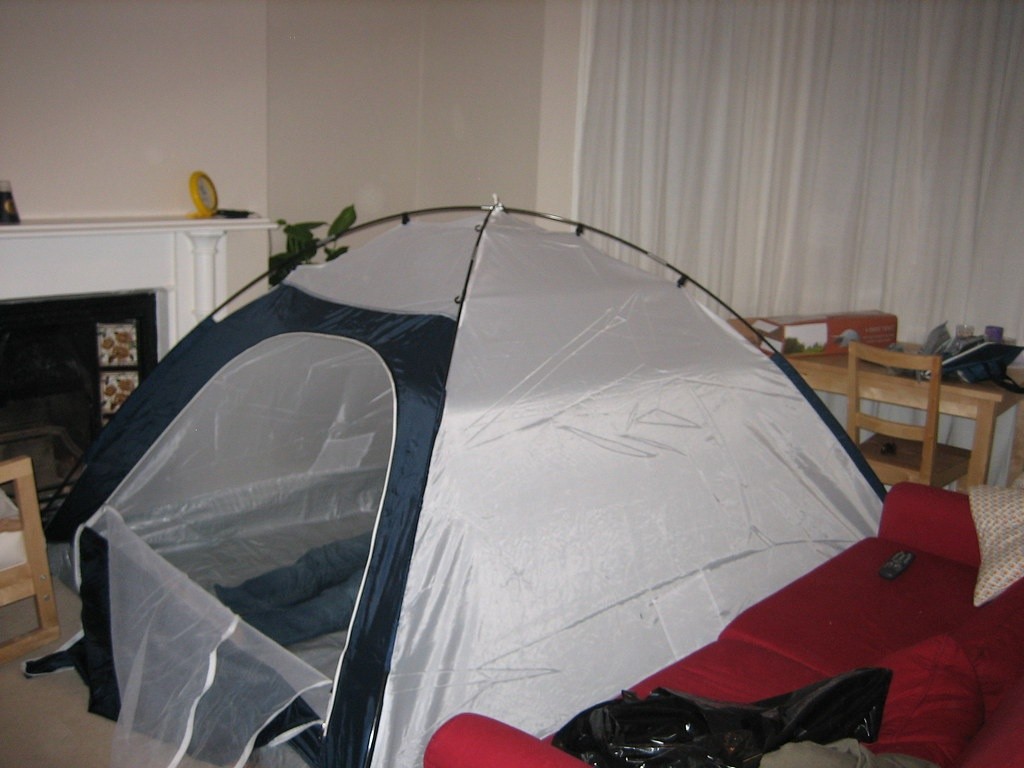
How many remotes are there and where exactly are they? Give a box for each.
[879,550,914,579]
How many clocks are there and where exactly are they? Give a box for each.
[189,172,219,218]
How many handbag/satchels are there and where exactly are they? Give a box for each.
[924,335,1024,394]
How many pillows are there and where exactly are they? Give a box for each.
[969,472,1024,606]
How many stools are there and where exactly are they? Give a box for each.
[0,445,60,664]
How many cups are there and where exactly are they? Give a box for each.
[955,323,975,338]
[983,325,1004,343]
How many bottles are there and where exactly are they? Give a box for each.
[0,178,20,226]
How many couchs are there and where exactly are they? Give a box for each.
[424,482,1024,768]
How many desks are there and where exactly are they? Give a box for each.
[785,347,1024,495]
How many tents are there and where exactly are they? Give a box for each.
[19,192,890,768]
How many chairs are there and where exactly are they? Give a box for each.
[845,340,973,493]
